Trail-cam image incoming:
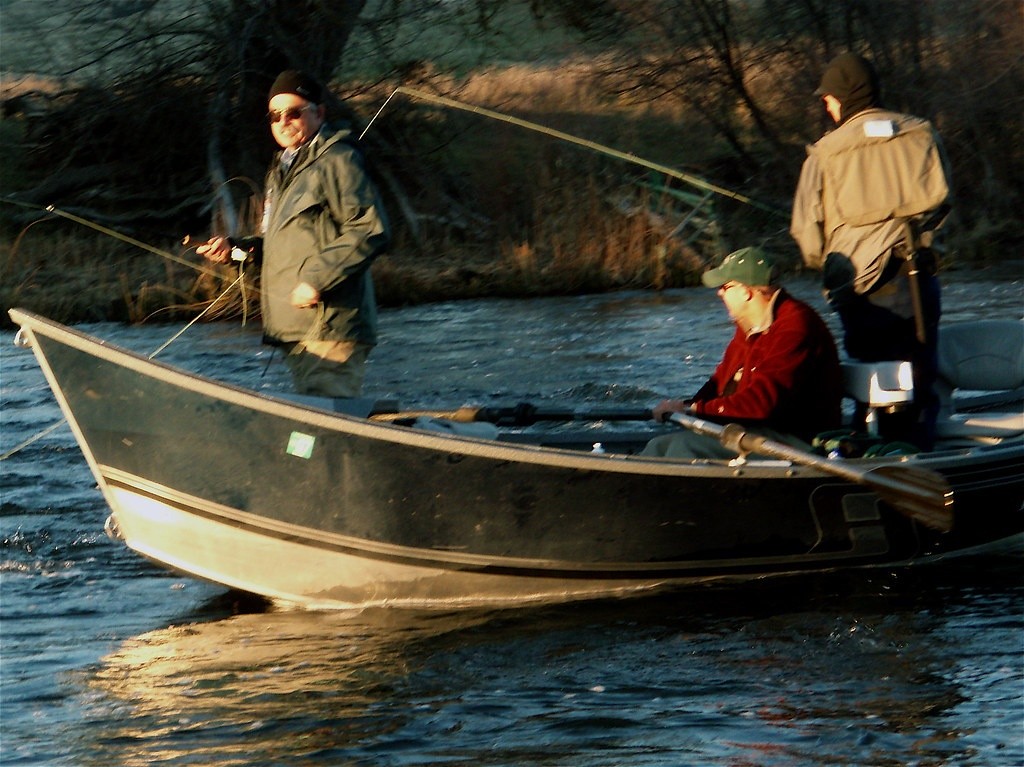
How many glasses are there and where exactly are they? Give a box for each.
[265,102,318,124]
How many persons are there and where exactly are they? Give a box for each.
[195,71,394,398]
[640,247,842,460]
[790,52,956,452]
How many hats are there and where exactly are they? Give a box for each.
[699,244,787,289]
[267,68,330,106]
[810,50,880,128]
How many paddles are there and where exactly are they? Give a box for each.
[662,411,954,530]
[366,408,654,421]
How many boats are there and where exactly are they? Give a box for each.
[7,306,1024,611]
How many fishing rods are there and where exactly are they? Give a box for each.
[397,87,791,221]
[0,201,209,264]
